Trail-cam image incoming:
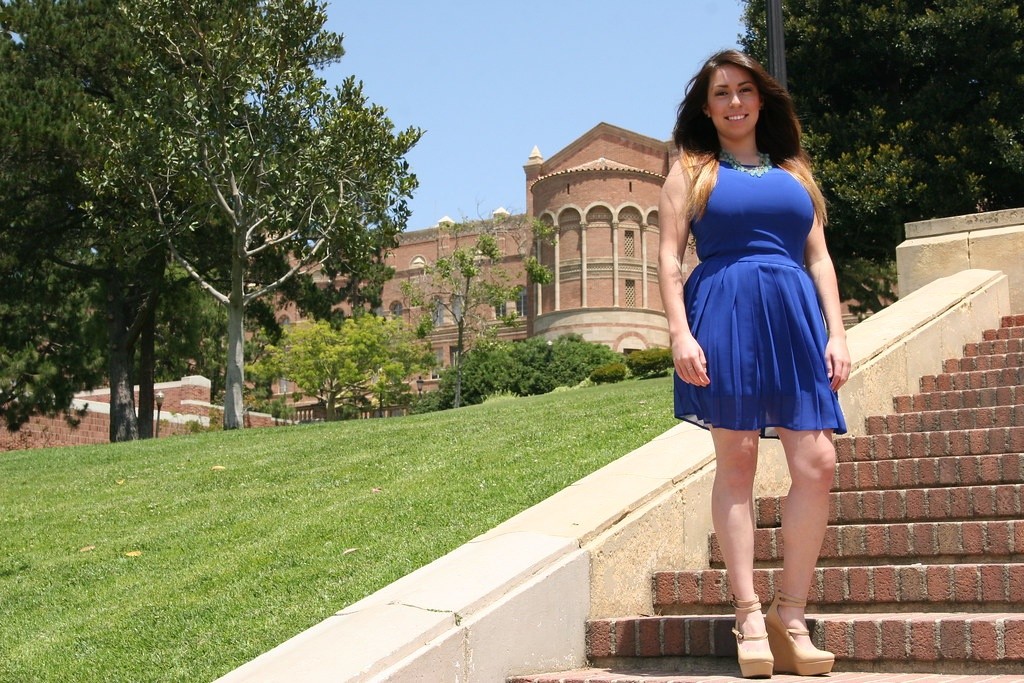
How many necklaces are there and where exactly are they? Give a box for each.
[719,148,771,177]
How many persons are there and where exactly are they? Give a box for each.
[658,50,851,677]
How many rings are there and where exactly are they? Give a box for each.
[688,367,693,371]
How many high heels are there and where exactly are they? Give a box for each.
[729,594,774,677]
[765,575,835,675]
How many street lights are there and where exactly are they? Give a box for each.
[416,376,424,399]
[155,390,165,437]
